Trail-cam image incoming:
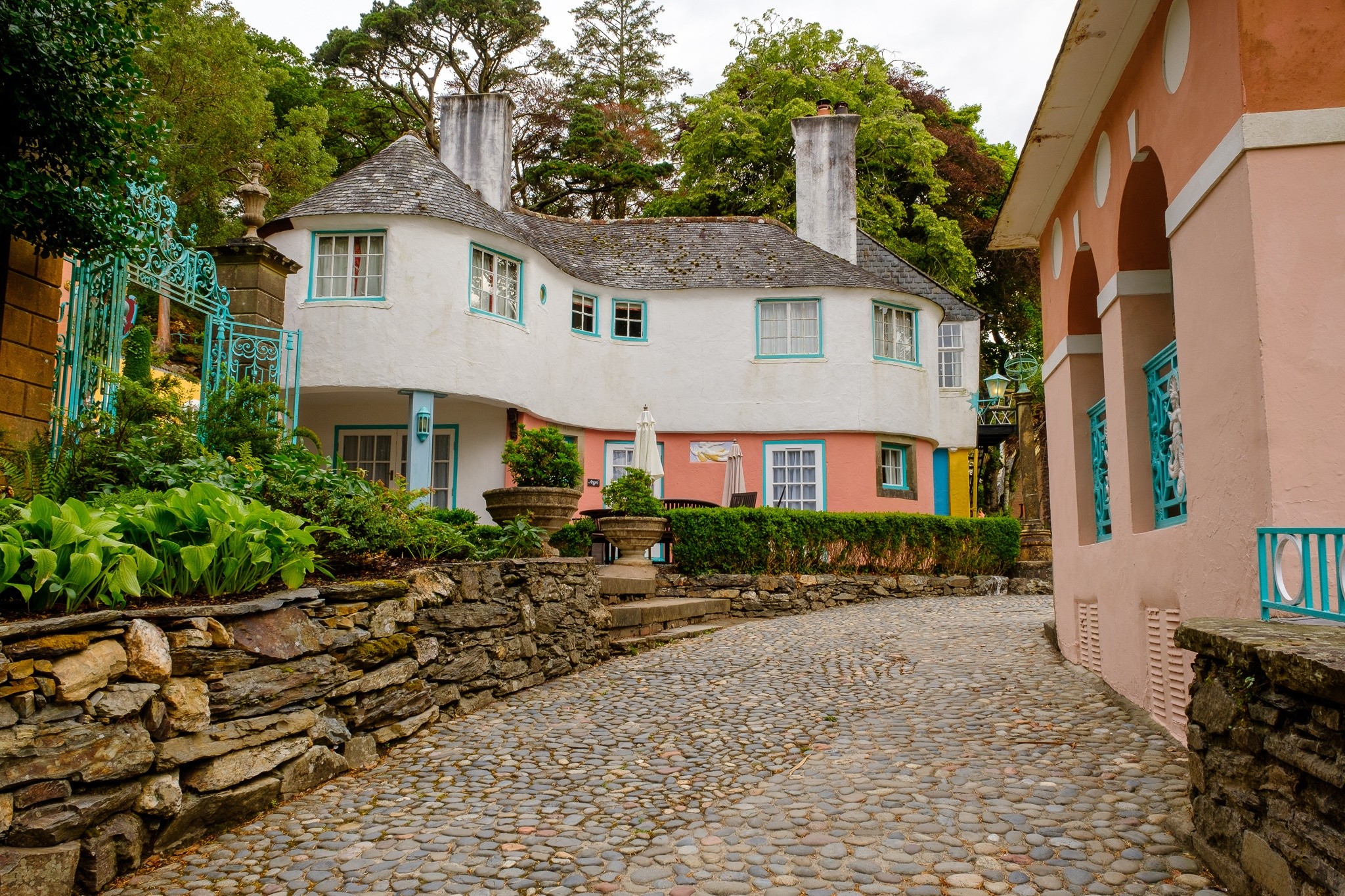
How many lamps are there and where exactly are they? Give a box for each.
[416,407,431,441]
[966,368,1010,415]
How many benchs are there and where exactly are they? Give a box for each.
[579,491,758,565]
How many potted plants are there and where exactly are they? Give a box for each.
[482,422,585,557]
[599,467,668,565]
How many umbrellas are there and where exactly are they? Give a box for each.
[720,438,747,509]
[630,404,665,498]
